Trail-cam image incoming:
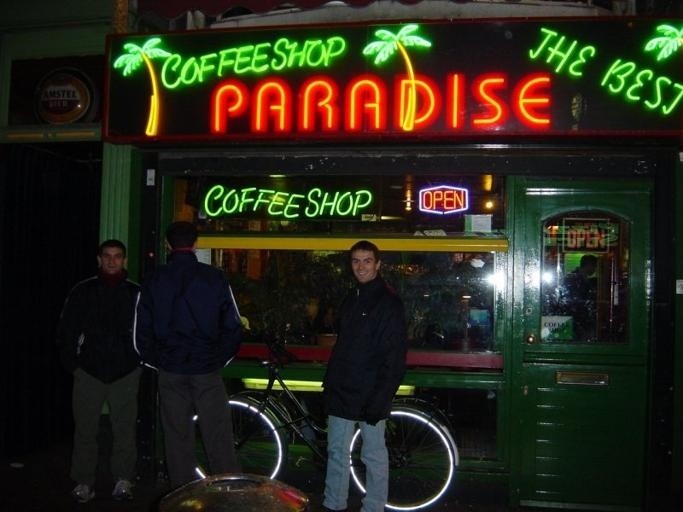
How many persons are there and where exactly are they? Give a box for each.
[50,239,143,504]
[316,239,408,511]
[132,220,247,494]
[559,253,599,320]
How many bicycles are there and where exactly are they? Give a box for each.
[193,361,460,511]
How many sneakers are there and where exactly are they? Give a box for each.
[110,477,137,504]
[69,482,96,503]
